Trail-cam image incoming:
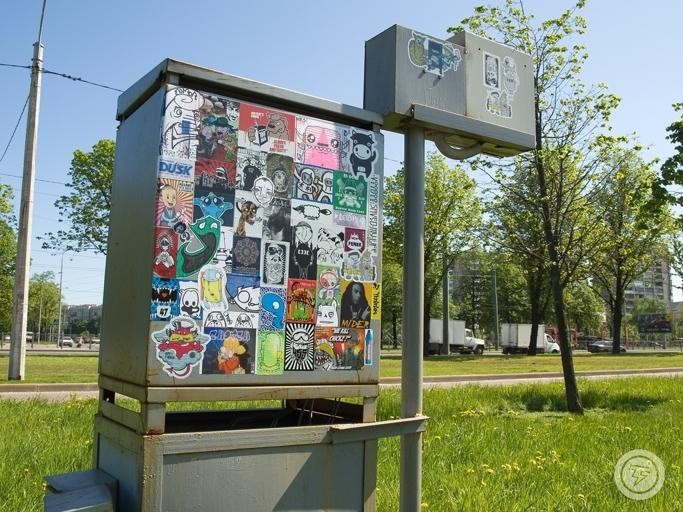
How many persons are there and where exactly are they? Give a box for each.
[241,158,263,191]
[341,281,371,328]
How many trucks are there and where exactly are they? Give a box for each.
[501,323,560,354]
[428,318,485,355]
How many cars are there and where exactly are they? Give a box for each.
[26,332,33,341]
[84,337,100,344]
[589,341,627,352]
[60,336,72,347]
